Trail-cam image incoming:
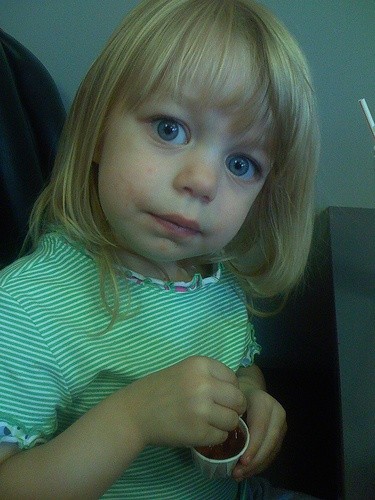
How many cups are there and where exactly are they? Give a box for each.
[192,417,250,478]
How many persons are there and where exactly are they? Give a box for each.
[0,0,321,500]
